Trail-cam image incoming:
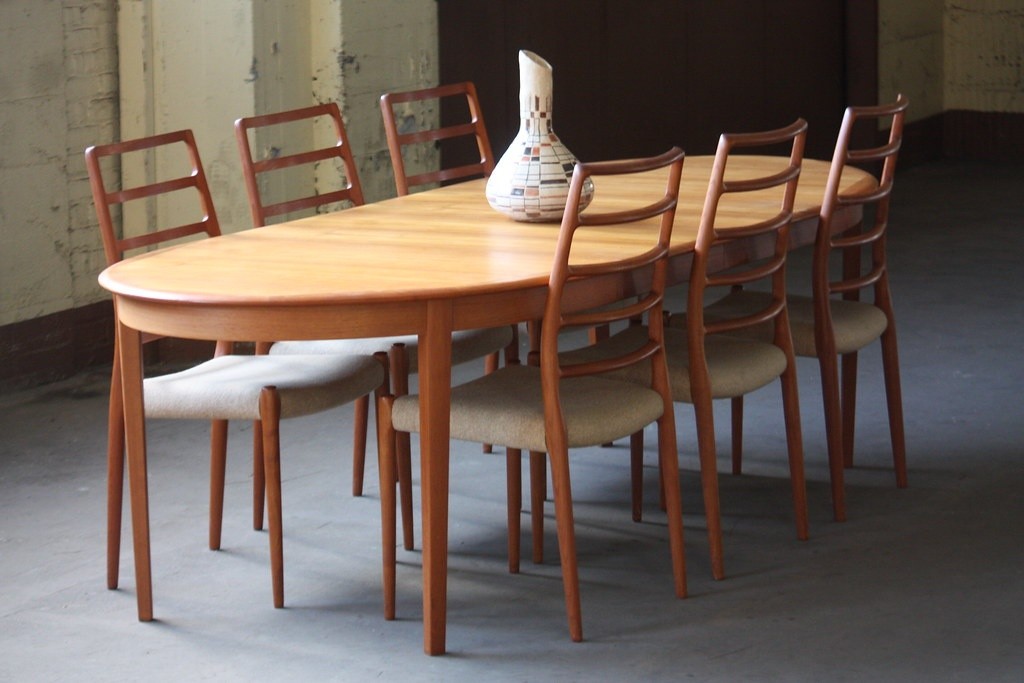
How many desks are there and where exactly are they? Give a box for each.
[99,154,879,656]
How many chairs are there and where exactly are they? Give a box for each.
[237,103,525,551]
[662,94,907,524]
[532,117,811,580]
[394,145,688,642]
[83,129,398,623]
[378,84,501,453]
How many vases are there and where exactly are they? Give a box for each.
[483,49,597,226]
